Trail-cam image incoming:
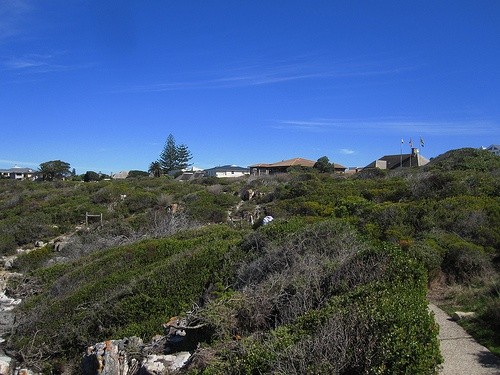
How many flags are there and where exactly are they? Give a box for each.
[409,138,413,148]
[420,137,424,147]
[401,138,404,144]
